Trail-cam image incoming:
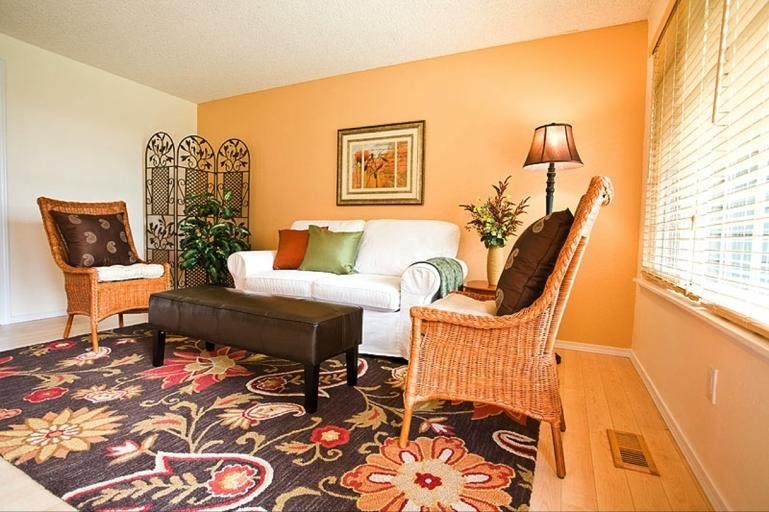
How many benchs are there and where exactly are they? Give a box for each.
[148,285,363,413]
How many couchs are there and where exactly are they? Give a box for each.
[226,218,468,362]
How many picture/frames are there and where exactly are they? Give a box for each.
[335,120,425,207]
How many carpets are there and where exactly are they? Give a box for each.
[0,323,540,512]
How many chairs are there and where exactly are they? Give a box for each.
[399,175,613,479]
[38,196,171,353]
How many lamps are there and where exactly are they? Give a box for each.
[522,123,584,217]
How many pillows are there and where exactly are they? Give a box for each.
[298,225,364,274]
[50,211,145,268]
[273,226,328,270]
[495,209,574,316]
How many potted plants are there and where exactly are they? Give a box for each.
[460,174,529,287]
[180,188,251,287]
